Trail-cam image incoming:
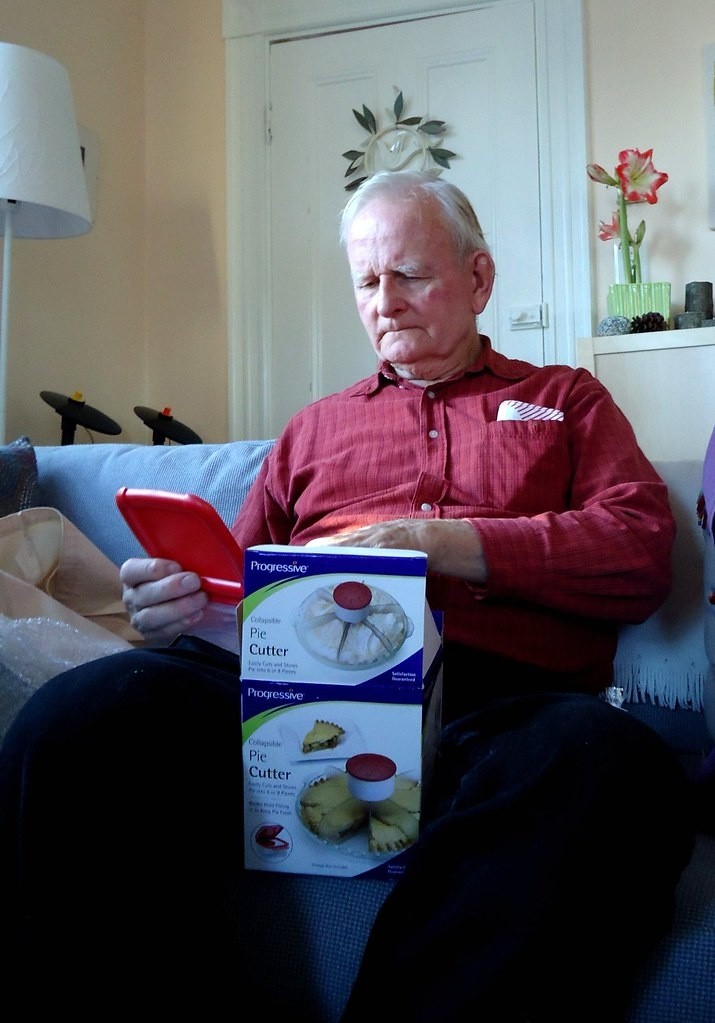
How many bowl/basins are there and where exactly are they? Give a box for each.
[293,765,422,859]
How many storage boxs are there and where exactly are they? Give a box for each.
[235,543,444,881]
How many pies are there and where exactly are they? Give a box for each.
[300,771,420,853]
[302,718,346,752]
[300,582,405,664]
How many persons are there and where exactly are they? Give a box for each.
[0,169,679,1023]
[697,425,715,740]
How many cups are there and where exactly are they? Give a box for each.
[673,310,703,329]
[685,281,713,320]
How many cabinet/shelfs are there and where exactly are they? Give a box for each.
[575,327,715,459]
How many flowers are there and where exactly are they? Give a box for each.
[585,149,671,283]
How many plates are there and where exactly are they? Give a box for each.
[277,715,366,762]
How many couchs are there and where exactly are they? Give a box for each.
[0,441,715,1023]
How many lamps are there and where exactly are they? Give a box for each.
[0,42,94,445]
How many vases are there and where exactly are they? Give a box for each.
[606,283,670,328]
